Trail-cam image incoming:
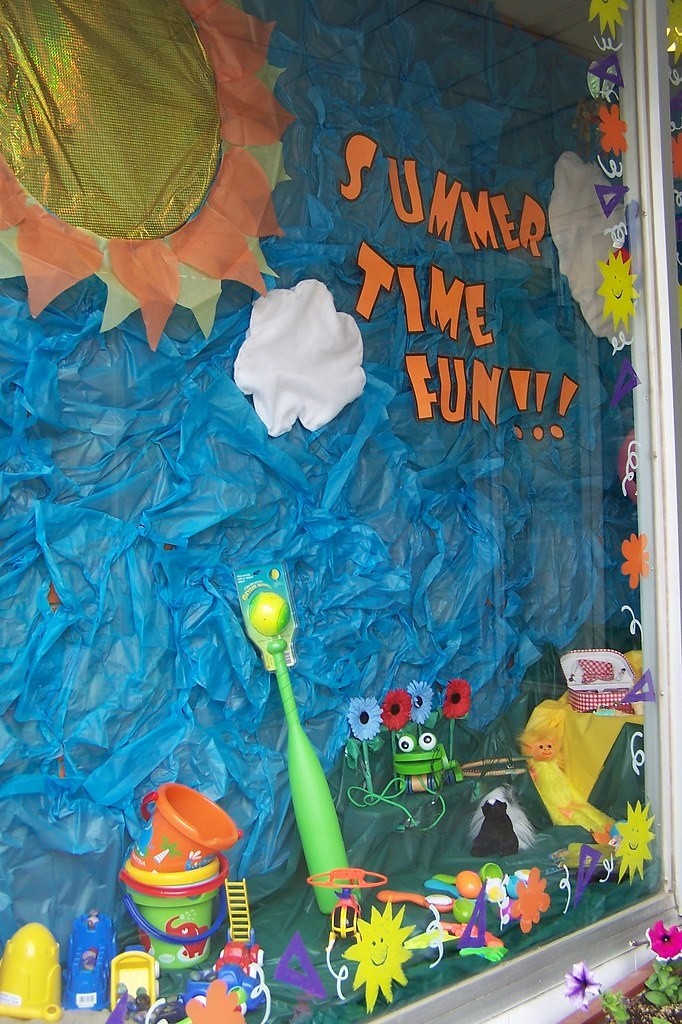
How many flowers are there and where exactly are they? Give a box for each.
[381,689,412,791]
[564,960,630,1024]
[644,920,682,1006]
[346,696,384,799]
[437,679,471,760]
[407,679,437,738]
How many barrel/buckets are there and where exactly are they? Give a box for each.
[130,783,243,873]
[117,852,235,968]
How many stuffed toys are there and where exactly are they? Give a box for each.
[518,723,621,846]
[467,784,538,857]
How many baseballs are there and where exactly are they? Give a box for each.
[250,591,291,638]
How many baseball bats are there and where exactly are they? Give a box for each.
[265,639,363,916]
